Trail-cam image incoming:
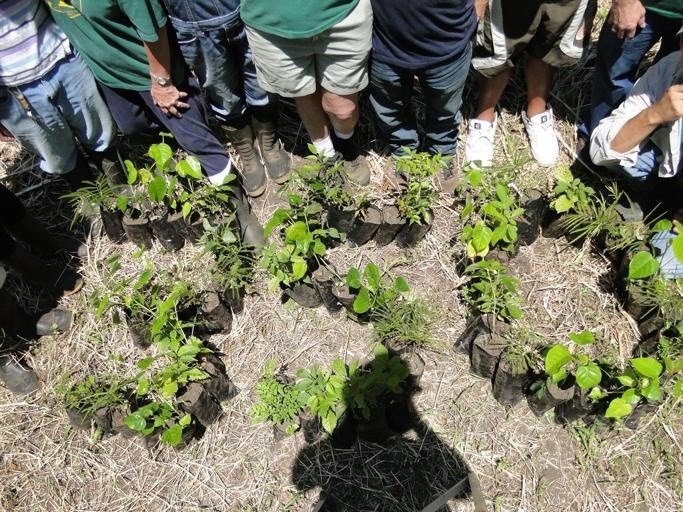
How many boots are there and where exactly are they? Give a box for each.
[222,122,267,197]
[207,167,264,259]
[249,118,292,184]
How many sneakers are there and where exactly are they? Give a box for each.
[331,129,370,185]
[464,111,497,172]
[520,103,559,168]
[430,156,459,196]
[589,102,612,138]
[382,155,411,195]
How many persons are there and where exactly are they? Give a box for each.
[240,0,374,190]
[45,0,268,257]
[588,24,683,182]
[577,0,683,137]
[0,288,76,397]
[370,0,488,194]
[0,183,88,297]
[465,0,597,174]
[0,0,126,198]
[163,0,293,198]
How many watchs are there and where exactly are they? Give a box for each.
[149,71,171,86]
